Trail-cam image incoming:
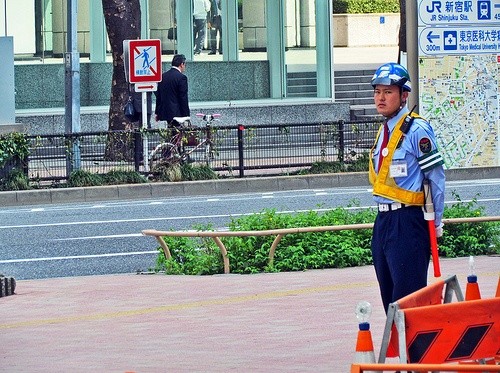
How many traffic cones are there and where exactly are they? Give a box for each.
[351,322,378,373]
[465,275,482,301]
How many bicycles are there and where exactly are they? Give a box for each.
[149,112,223,172]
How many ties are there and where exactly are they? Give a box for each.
[378,121,388,174]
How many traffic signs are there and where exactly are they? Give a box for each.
[418,26,500,56]
[416,0,500,26]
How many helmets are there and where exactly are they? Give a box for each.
[371,63,412,92]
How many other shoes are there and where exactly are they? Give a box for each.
[219,51,222,54]
[208,51,216,55]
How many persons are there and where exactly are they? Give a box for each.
[193,0,222,55]
[368,63,445,315]
[153,55,195,167]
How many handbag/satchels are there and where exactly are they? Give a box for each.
[170,120,199,147]
[168,25,177,40]
[124,93,138,123]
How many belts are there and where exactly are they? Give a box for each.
[378,202,409,212]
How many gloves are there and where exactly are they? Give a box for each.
[436,224,444,237]
